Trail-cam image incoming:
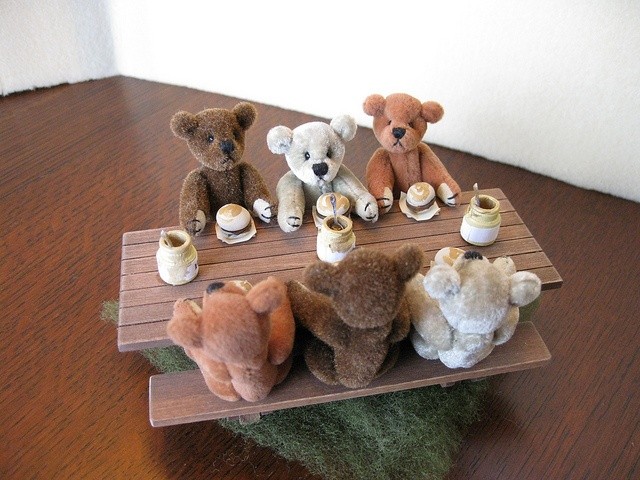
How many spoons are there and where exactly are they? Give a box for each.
[329,193,344,231]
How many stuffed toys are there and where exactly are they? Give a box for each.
[167,274,297,404]
[363,92,462,211]
[284,241,423,389]
[170,101,279,232]
[404,247,542,369]
[267,112,379,233]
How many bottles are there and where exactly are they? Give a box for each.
[155,228,201,284]
[459,195,502,248]
[315,214,356,266]
